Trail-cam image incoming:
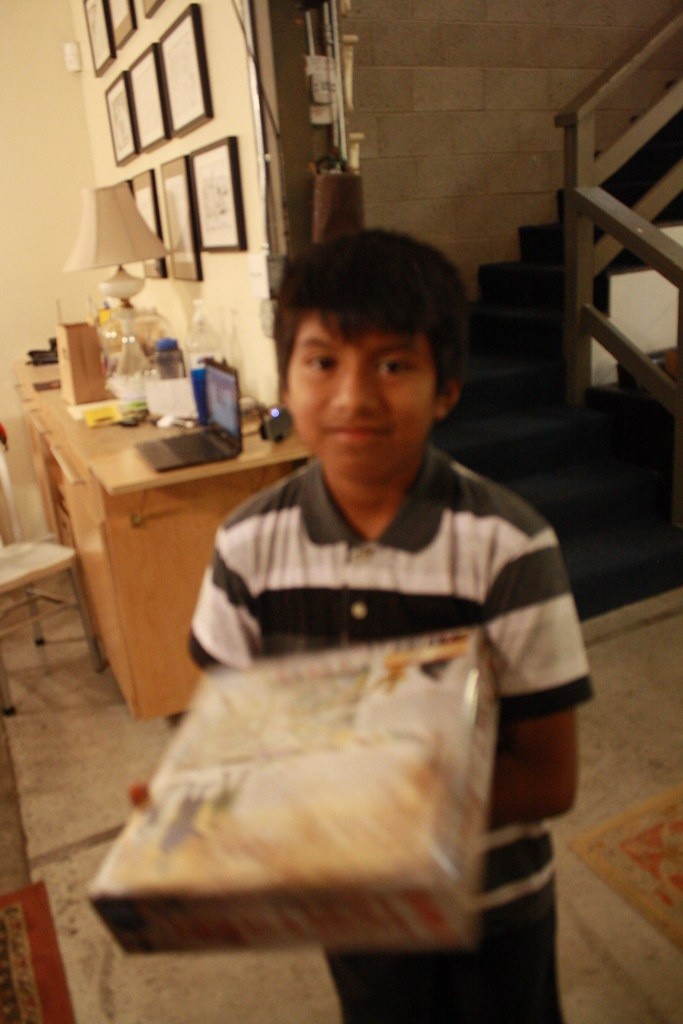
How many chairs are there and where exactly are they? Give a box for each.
[0,444,106,715]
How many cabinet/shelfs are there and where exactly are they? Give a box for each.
[12,370,312,716]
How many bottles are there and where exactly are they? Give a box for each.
[103,311,159,402]
[155,338,188,380]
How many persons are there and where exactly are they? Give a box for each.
[190,230,597,1015]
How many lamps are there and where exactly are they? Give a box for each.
[62,179,169,384]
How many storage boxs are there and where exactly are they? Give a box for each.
[87,625,505,956]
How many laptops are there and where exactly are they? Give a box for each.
[134,359,242,473]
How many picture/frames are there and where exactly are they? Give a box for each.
[188,135,249,252]
[160,2,214,141]
[160,154,201,281]
[128,40,172,154]
[83,0,116,80]
[104,70,140,167]
[105,0,138,51]
[130,170,169,281]
